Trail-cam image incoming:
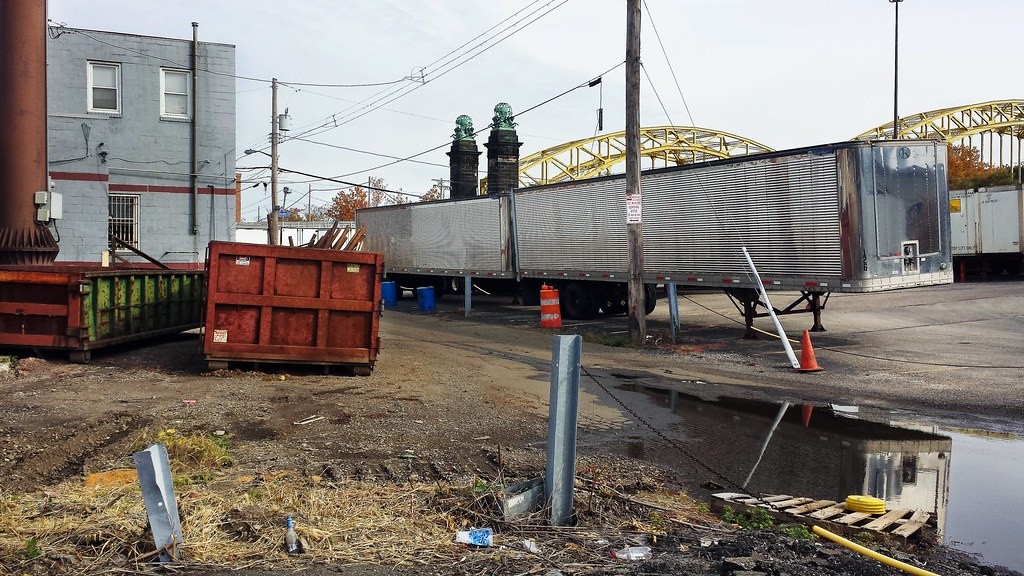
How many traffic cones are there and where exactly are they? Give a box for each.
[796,330,823,372]
[803,404,813,427]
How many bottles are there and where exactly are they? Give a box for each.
[612,547,652,560]
[286,516,298,553]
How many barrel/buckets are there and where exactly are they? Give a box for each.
[381,281,397,309]
[417,286,436,312]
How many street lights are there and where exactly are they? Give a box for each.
[243,149,278,244]
[890,0,901,140]
